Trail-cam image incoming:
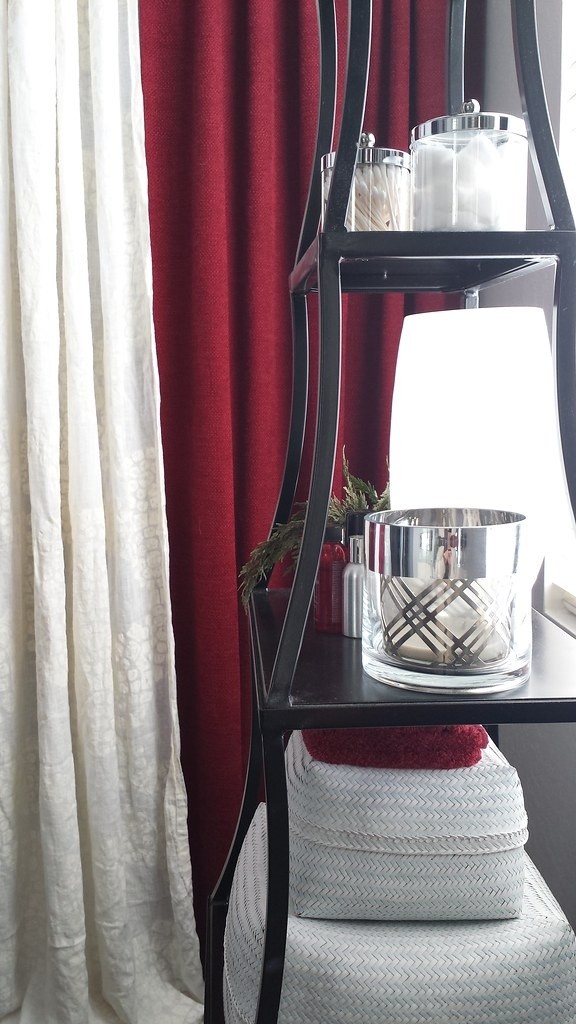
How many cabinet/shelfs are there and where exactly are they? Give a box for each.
[193,0,575,1024]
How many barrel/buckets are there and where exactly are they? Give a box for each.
[361,507,534,695]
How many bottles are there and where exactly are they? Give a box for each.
[409,98,528,231]
[320,131,408,230]
[311,512,362,640]
